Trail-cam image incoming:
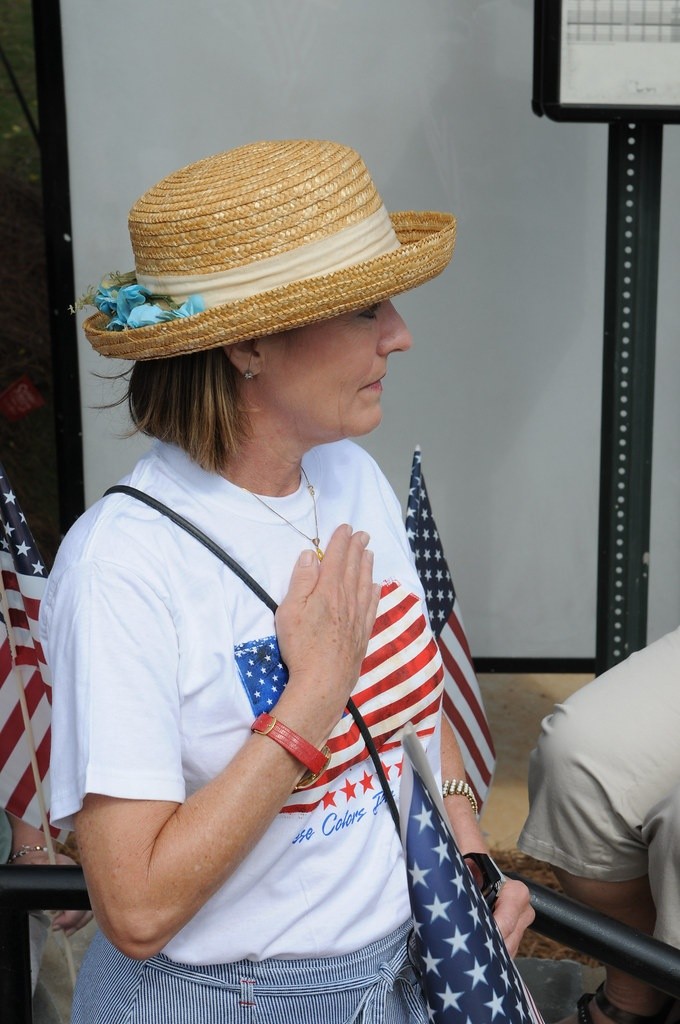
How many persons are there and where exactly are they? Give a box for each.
[0,806,94,937]
[517,631,680,1024]
[38,141,534,1024]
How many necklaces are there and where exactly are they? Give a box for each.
[247,465,325,561]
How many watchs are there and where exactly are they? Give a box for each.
[250,712,332,791]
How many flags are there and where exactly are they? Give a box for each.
[405,450,498,812]
[0,477,68,846]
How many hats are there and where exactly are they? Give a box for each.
[81,140,457,362]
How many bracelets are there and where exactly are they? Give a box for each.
[9,846,60,864]
[440,780,480,817]
